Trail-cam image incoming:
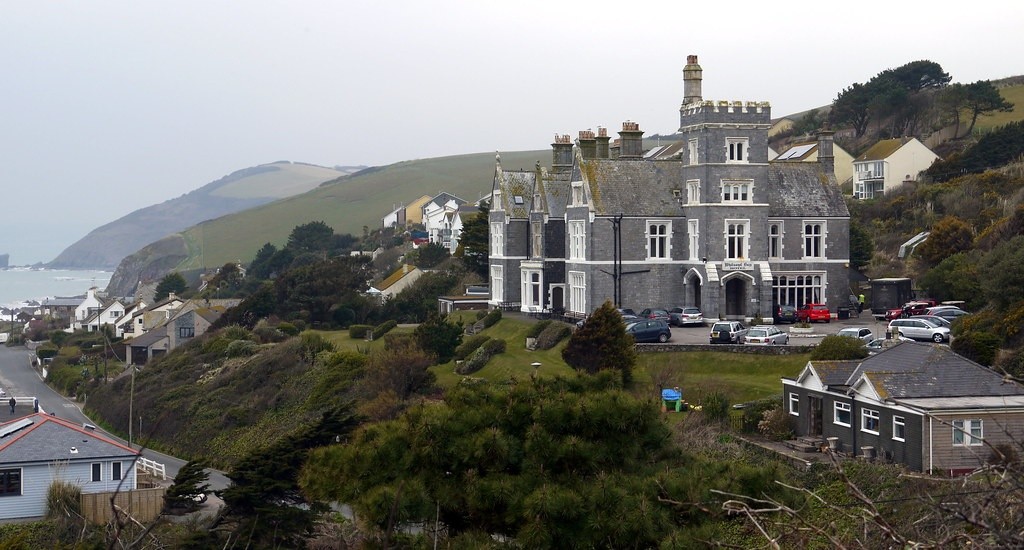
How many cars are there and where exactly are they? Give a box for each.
[863,336,916,349]
[836,327,874,345]
[888,319,951,343]
[932,309,972,321]
[177,494,207,509]
[797,304,831,324]
[669,306,703,327]
[575,308,672,343]
[922,306,961,320]
[910,315,951,327]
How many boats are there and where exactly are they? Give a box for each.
[661,388,682,410]
[732,403,748,410]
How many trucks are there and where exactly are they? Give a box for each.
[871,278,916,320]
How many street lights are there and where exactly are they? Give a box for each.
[128,366,141,448]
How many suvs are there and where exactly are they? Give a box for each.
[885,299,936,321]
[708,320,748,344]
[774,303,798,325]
[640,308,671,325]
[745,324,789,346]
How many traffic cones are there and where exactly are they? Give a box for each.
[834,312,839,320]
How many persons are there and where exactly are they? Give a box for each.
[9,397,16,414]
[858,292,866,312]
